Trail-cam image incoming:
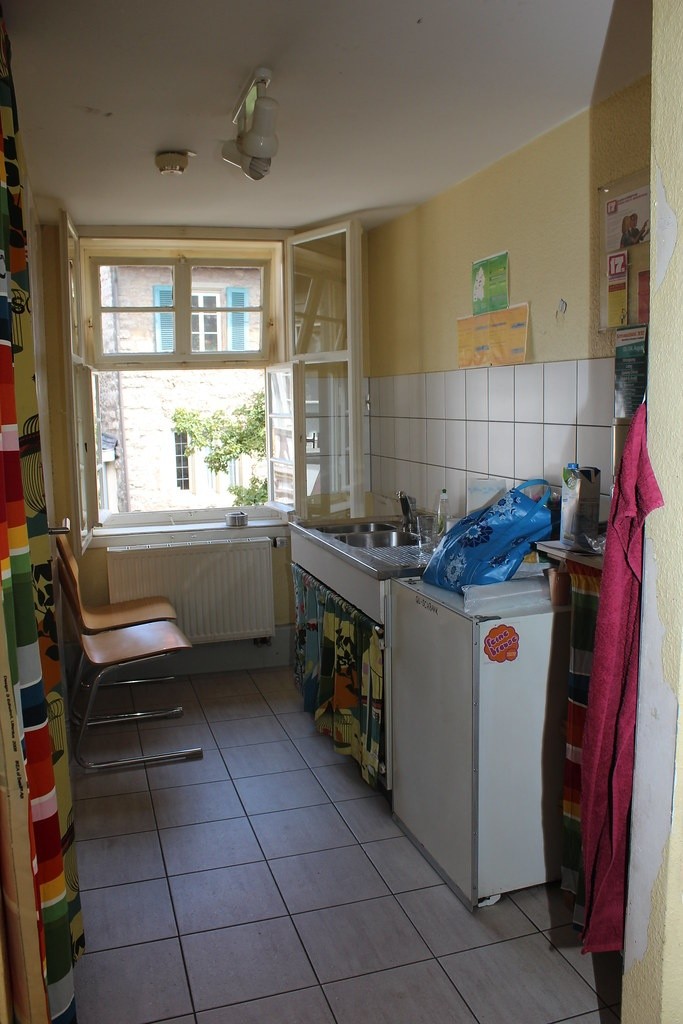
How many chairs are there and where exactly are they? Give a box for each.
[53,530,203,772]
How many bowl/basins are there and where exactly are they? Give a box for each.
[225,512,248,526]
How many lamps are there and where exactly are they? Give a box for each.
[217,65,280,182]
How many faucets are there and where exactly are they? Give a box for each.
[397,490,418,533]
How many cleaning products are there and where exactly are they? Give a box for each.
[437,488,451,529]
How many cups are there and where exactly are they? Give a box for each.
[417,515,437,551]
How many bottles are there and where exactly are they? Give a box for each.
[437,489,452,537]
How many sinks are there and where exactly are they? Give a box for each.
[335,532,433,550]
[315,522,398,534]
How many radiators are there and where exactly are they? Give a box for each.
[106,535,287,647]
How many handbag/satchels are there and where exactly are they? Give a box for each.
[421,479,562,592]
